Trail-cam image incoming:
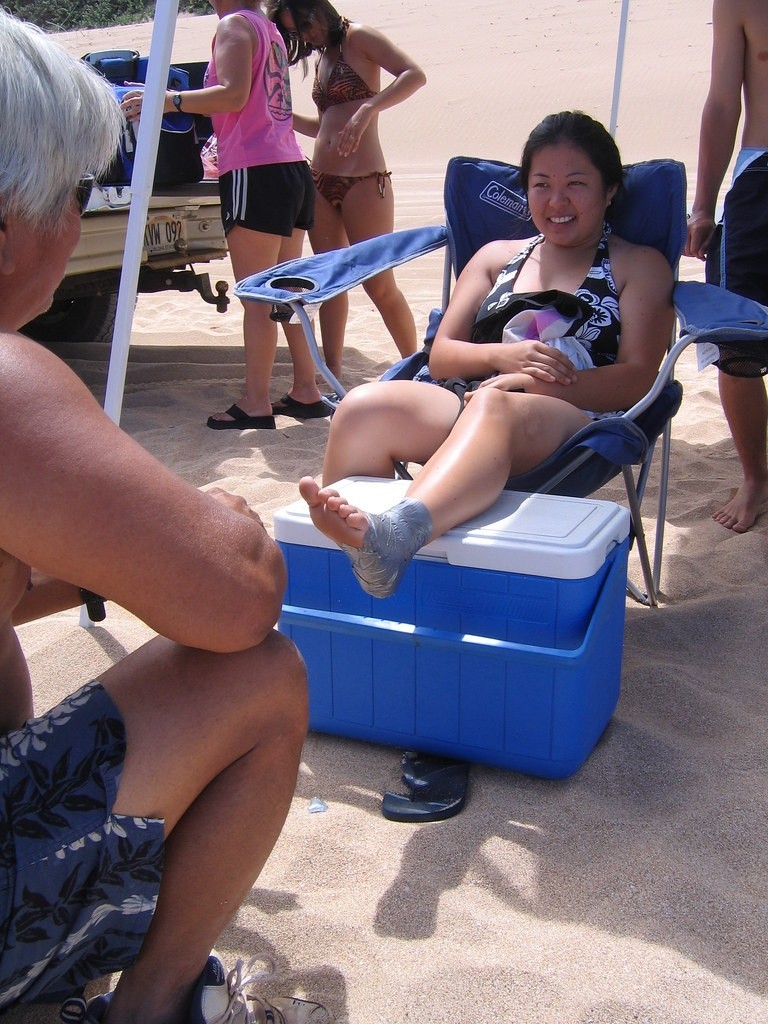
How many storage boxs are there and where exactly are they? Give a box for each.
[268,475,632,785]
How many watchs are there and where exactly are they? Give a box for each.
[173,92,183,112]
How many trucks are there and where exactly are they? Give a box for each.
[17,179,228,343]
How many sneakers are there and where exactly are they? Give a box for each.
[57,954,332,1024]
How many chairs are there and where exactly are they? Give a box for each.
[233,152,768,610]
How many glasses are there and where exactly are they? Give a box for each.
[286,8,316,40]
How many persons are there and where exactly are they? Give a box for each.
[684,0,768,532]
[298,107,675,598]
[116,0,333,430]
[267,0,427,383]
[0,11,328,1024]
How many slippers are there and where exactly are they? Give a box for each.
[207,403,276,429]
[271,391,331,418]
[381,750,470,822]
